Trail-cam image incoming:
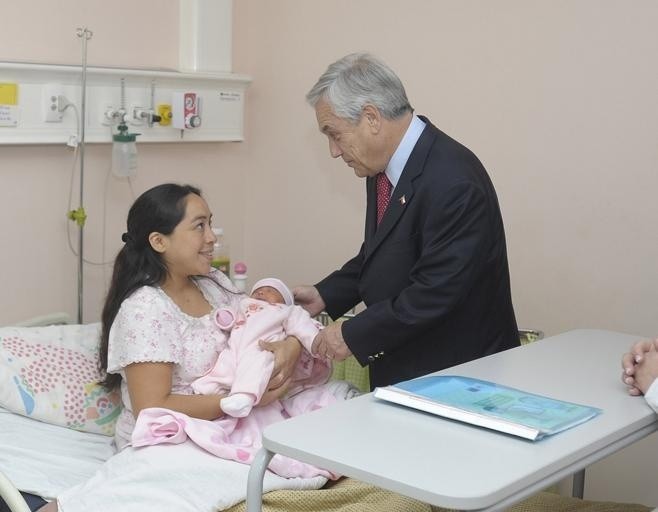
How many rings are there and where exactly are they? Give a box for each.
[326,354,332,360]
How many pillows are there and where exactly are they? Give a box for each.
[0,318,134,438]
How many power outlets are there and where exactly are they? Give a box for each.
[39,87,69,124]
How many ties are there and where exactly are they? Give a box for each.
[372,169,394,233]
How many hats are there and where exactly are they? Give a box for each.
[250,277,296,307]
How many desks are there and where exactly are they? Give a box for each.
[243,321,657,512]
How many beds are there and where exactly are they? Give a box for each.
[0,310,657,512]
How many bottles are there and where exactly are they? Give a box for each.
[110,134,140,178]
[209,228,230,284]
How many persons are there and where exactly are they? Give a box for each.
[622,336,658,415]
[98,184,321,450]
[192,276,319,418]
[289,50,521,393]
